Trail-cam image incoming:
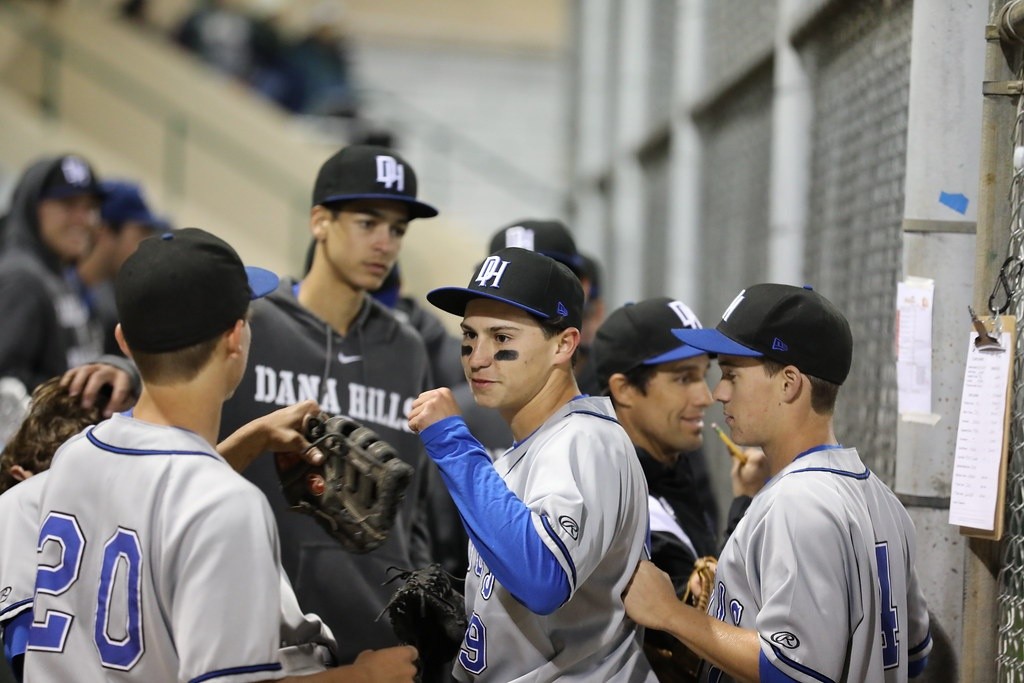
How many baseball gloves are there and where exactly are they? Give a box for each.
[382,563,486,677]
[265,405,423,561]
[1,371,140,492]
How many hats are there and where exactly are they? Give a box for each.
[116,226,279,357]
[99,176,173,232]
[426,247,586,331]
[41,163,109,203]
[489,217,591,280]
[310,145,440,220]
[591,296,716,397]
[670,283,853,386]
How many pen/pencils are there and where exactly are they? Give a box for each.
[710,421,748,466]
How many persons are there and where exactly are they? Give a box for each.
[206,142,465,683]
[407,220,660,682]
[0,355,142,683]
[0,153,113,395]
[57,177,171,367]
[621,282,934,683]
[22,226,420,683]
[592,295,715,683]
